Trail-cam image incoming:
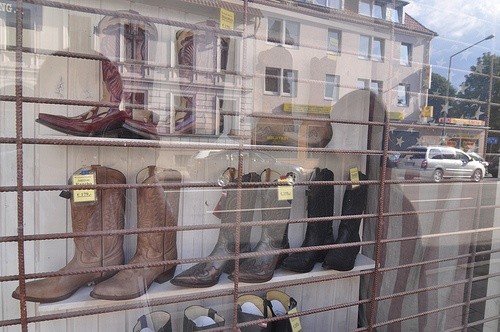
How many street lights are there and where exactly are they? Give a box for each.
[443,34,496,144]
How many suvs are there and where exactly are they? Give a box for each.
[396,144,487,184]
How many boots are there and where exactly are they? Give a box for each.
[11,165,124,302]
[280,167,336,273]
[229,56,337,148]
[238,294,273,331]
[321,172,368,271]
[266,290,302,332]
[213,44,295,144]
[227,168,296,283]
[90,165,181,300]
[171,172,260,288]
[183,305,224,332]
[123,20,230,142]
[133,310,173,332]
[33,10,159,137]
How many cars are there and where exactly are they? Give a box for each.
[382,152,398,168]
[188,148,305,190]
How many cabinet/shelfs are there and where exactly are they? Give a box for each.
[1,139,380,332]
